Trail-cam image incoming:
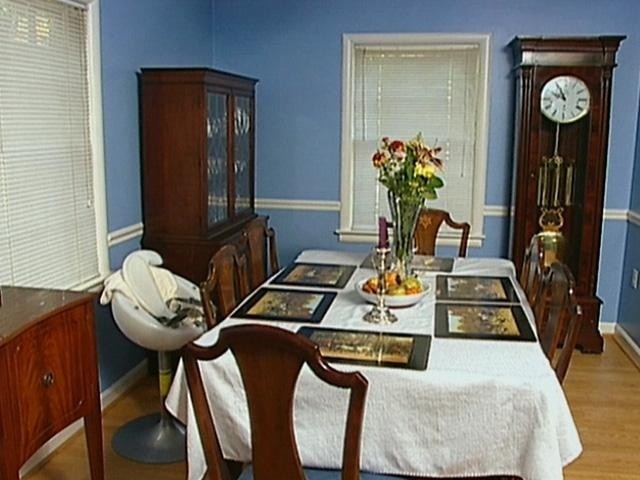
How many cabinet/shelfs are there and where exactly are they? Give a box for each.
[0,286,105,480]
[138,67,269,363]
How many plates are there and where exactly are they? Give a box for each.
[357,277,433,307]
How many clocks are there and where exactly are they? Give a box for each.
[507,34,627,353]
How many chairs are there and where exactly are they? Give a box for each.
[100,250,216,464]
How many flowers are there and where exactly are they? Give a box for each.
[373,132,444,267]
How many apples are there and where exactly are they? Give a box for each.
[362,269,424,297]
